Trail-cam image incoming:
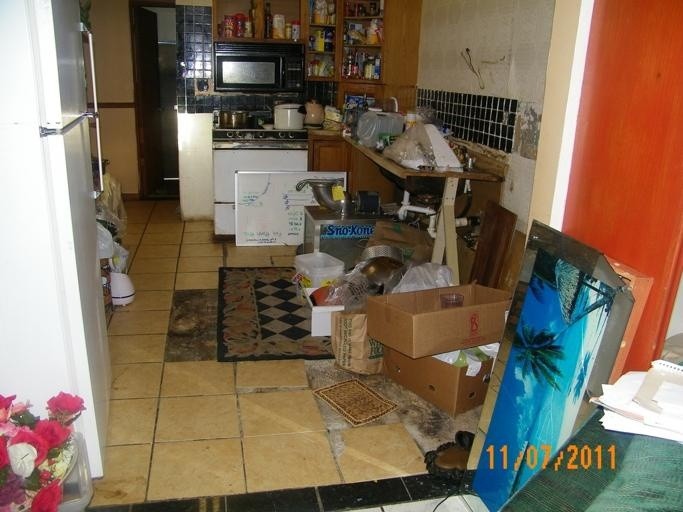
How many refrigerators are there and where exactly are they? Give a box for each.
[0,0,113,480]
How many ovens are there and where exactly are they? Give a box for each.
[214,142,308,239]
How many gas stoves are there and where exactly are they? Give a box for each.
[212,122,307,140]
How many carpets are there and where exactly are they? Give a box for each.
[216,266,337,363]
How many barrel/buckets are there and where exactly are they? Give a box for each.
[379,109,403,137]
[293,250,344,303]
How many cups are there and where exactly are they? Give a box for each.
[441,292,464,309]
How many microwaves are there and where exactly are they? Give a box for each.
[213,42,303,92]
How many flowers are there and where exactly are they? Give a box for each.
[0,390,88,512]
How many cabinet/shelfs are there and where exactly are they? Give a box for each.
[311,133,395,204]
[211,0,422,112]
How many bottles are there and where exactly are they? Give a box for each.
[405,111,416,131]
[264,3,300,39]
[362,92,368,113]
[343,1,383,81]
[308,0,335,78]
[305,100,325,125]
[451,142,475,171]
[217,8,255,38]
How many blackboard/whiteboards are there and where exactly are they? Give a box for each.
[234,170,347,247]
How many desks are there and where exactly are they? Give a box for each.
[496,360,683,512]
[341,128,508,205]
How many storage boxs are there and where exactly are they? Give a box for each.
[381,342,501,418]
[366,282,513,361]
[301,287,346,338]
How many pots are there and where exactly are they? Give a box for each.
[273,104,305,130]
[220,111,249,128]
